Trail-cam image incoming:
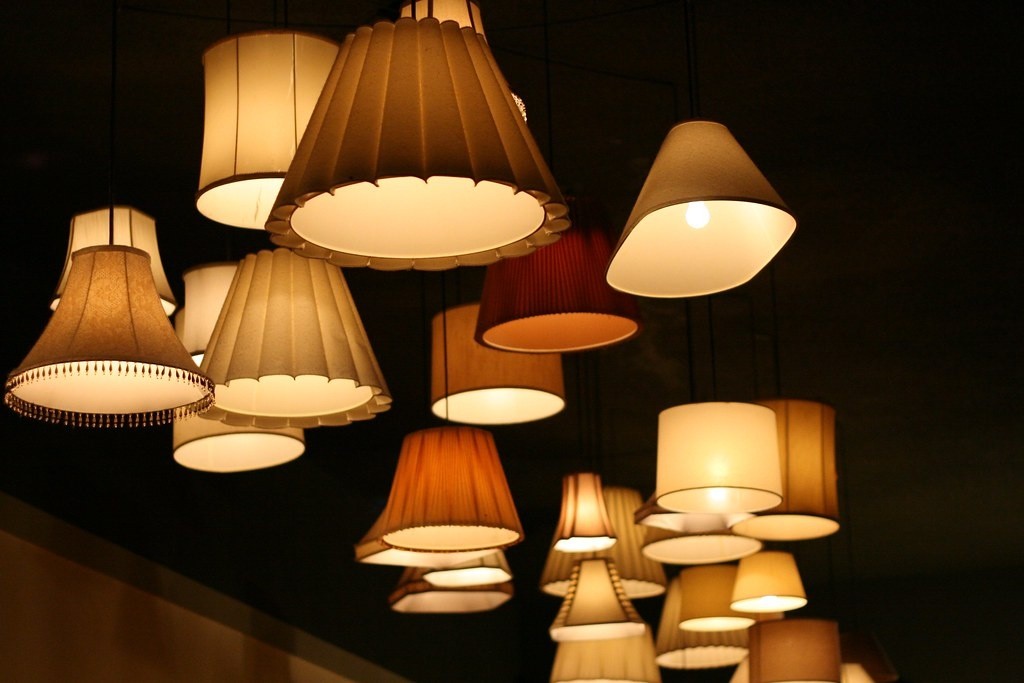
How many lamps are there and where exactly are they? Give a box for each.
[552,473,619,553]
[2,0,216,430]
[262,17,568,272]
[550,625,663,683]
[422,550,514,588]
[172,355,305,473]
[475,0,644,355]
[376,426,522,553]
[387,567,514,614]
[633,397,875,683]
[183,265,238,363]
[399,0,485,37]
[196,32,337,231]
[602,122,797,299]
[355,508,504,569]
[540,487,668,599]
[548,554,647,642]
[430,306,564,424]
[189,247,391,430]
[48,0,179,318]
